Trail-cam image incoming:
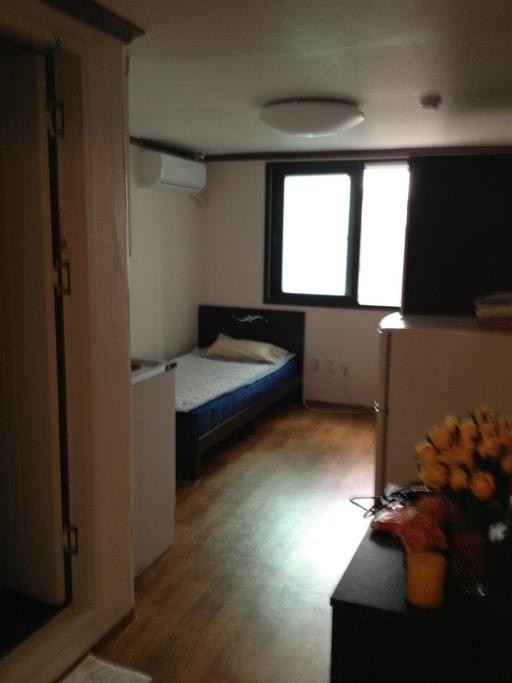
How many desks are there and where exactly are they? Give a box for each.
[330,517,511,683]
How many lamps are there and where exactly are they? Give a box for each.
[259,101,366,139]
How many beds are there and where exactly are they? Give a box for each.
[162,304,305,482]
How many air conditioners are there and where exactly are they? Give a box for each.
[132,149,207,197]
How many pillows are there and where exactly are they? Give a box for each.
[205,332,288,365]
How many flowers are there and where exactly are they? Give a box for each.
[410,404,512,543]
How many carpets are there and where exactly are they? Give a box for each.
[60,654,152,683]
